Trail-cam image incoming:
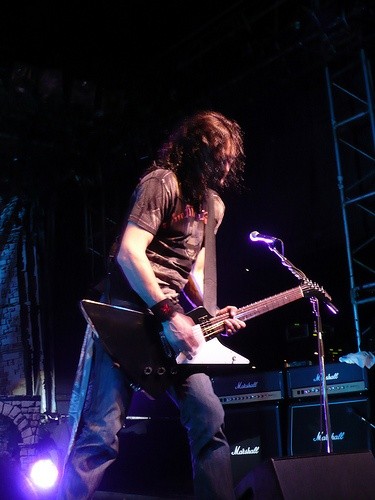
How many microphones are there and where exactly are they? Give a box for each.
[249,230,275,244]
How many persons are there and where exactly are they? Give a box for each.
[57,112,247,500]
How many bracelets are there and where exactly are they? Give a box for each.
[150,298,178,322]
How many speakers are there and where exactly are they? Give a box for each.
[220,404,281,488]
[290,398,375,459]
[232,451,375,500]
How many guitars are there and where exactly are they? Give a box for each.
[78,275,333,391]
